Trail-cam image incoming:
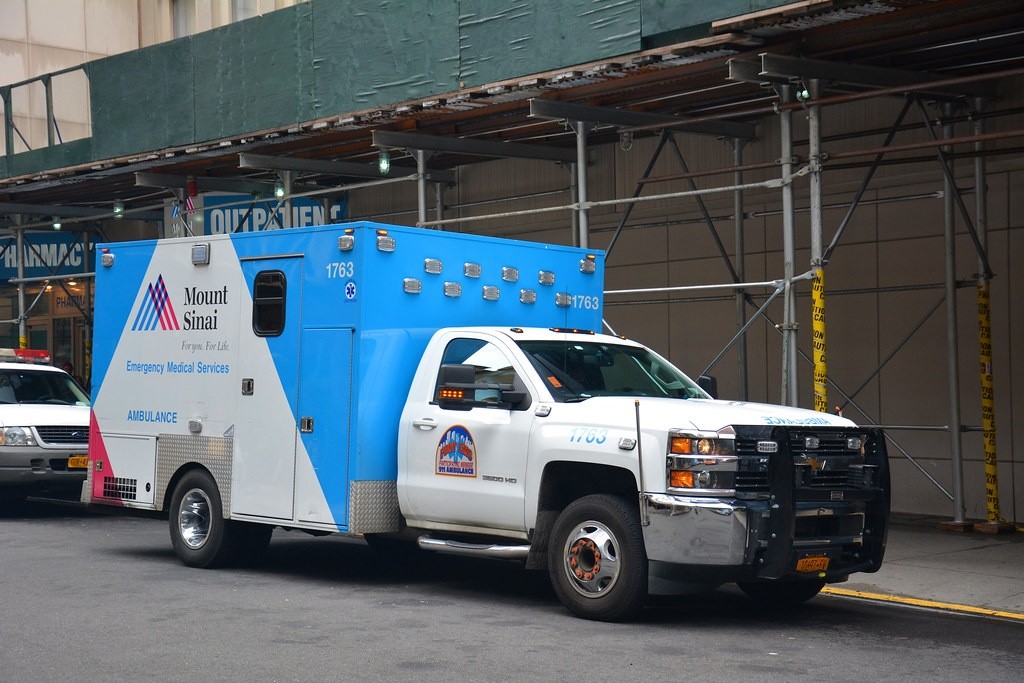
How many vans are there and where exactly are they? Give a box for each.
[0,346,92,502]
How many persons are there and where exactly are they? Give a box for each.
[61,360,82,387]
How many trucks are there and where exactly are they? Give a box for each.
[78,219,898,619]
